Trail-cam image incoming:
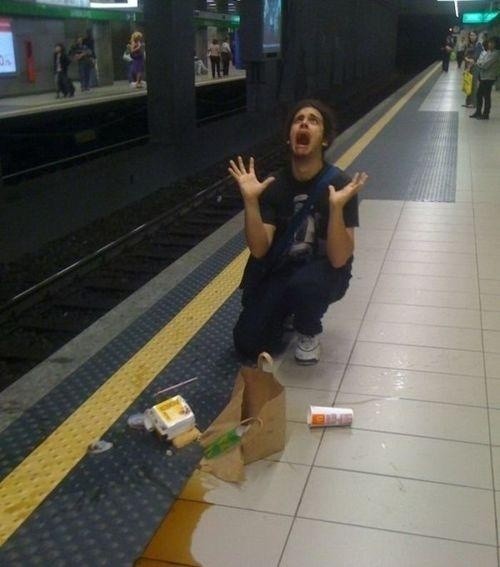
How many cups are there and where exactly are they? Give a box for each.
[306,405,354,429]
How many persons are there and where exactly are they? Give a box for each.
[194,50,209,75]
[441,29,499,120]
[220,36,233,77]
[227,98,369,365]
[126,42,145,86]
[52,42,76,99]
[75,36,96,91]
[128,30,145,88]
[208,38,222,80]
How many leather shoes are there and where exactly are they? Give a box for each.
[470,112,489,119]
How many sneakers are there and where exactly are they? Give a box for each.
[294,333,321,364]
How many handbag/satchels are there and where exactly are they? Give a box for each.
[241,256,271,291]
[462,72,473,96]
